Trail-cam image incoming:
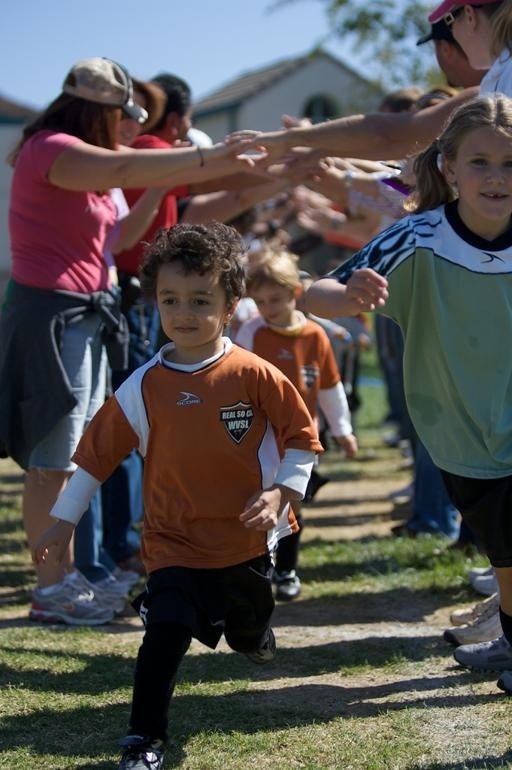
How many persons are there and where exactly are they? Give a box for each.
[0,0,512,693]
[32,223,327,770]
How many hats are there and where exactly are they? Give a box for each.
[414,0,498,47]
[63,56,149,124]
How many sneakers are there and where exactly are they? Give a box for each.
[272,568,302,602]
[243,625,277,665]
[28,533,149,626]
[116,731,168,770]
[381,411,512,699]
